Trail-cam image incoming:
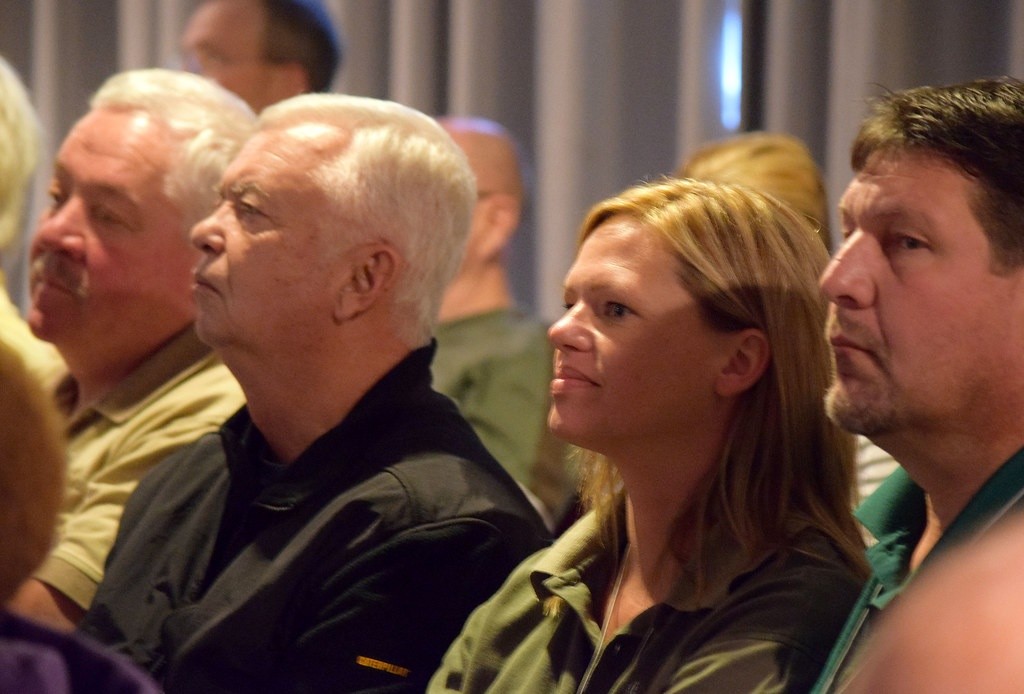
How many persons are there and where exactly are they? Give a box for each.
[69,93,556,693]
[173,0,345,122]
[814,67,1024,694]
[7,64,267,693]
[551,121,909,557]
[0,57,75,420]
[427,173,880,694]
[402,115,592,539]
[0,272,166,694]
